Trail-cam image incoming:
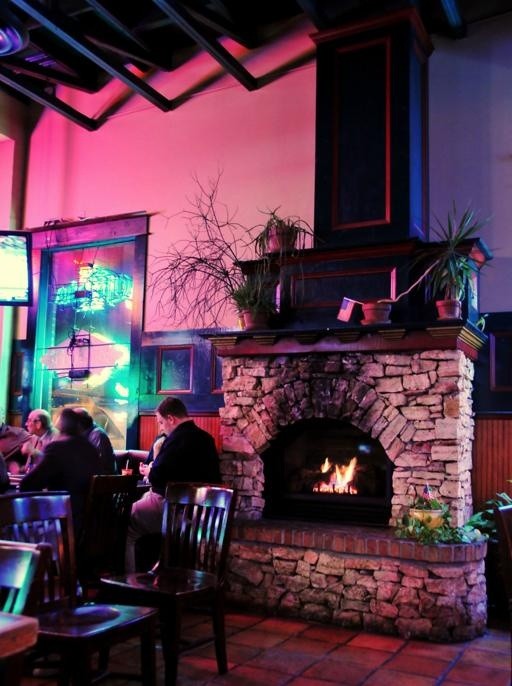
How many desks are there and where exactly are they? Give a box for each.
[0,608,43,659]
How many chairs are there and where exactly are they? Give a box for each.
[77,470,142,547]
[0,494,168,686]
[0,536,59,626]
[489,501,512,567]
[96,479,240,686]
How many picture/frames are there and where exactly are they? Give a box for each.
[29,232,152,452]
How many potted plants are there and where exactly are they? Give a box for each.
[138,170,248,332]
[390,474,512,544]
[230,272,286,332]
[239,205,321,255]
[403,198,497,319]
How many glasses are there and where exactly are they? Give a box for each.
[28,417,40,422]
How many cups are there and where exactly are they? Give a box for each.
[121,469,133,476]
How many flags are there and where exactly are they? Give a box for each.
[337,297,355,322]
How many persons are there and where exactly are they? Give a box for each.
[0,397,221,577]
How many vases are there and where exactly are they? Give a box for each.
[359,302,391,322]
